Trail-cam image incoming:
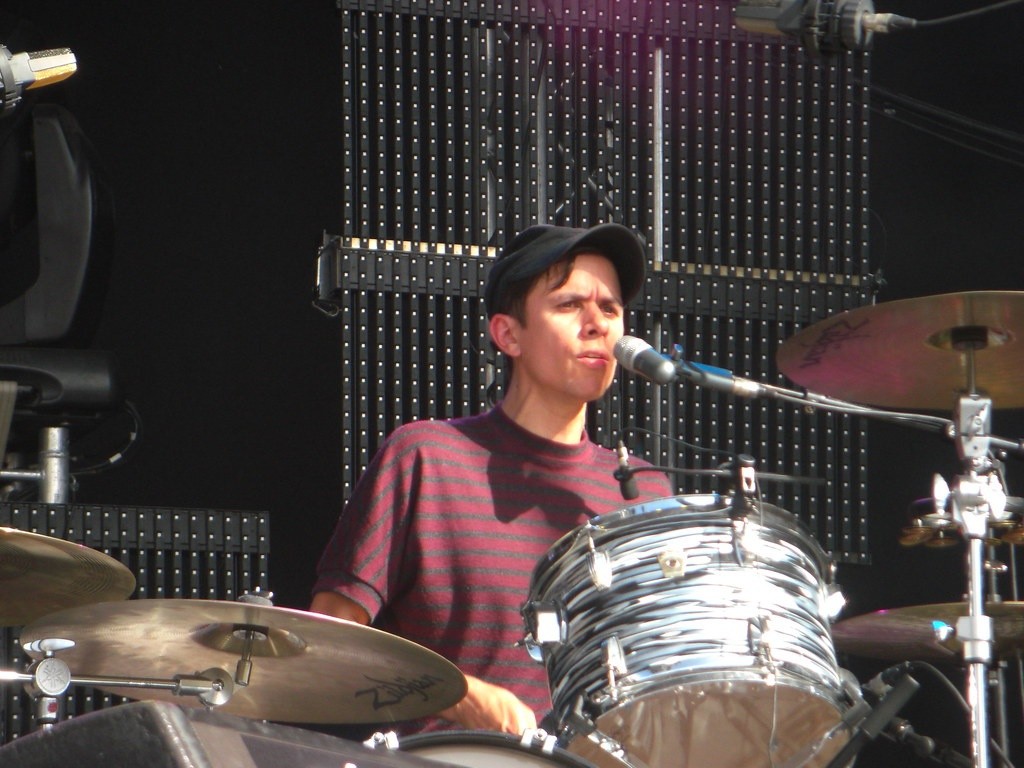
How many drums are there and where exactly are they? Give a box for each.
[354,723,588,767]
[519,490,863,766]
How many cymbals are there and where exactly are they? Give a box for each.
[776,291,1024,404]
[1,525,136,629]
[16,592,463,728]
[826,603,1024,659]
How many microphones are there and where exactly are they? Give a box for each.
[860,659,913,705]
[612,335,676,385]
[616,439,640,501]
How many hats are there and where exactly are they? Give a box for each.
[484,224,646,321]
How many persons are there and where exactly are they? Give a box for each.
[307,224,678,736]
